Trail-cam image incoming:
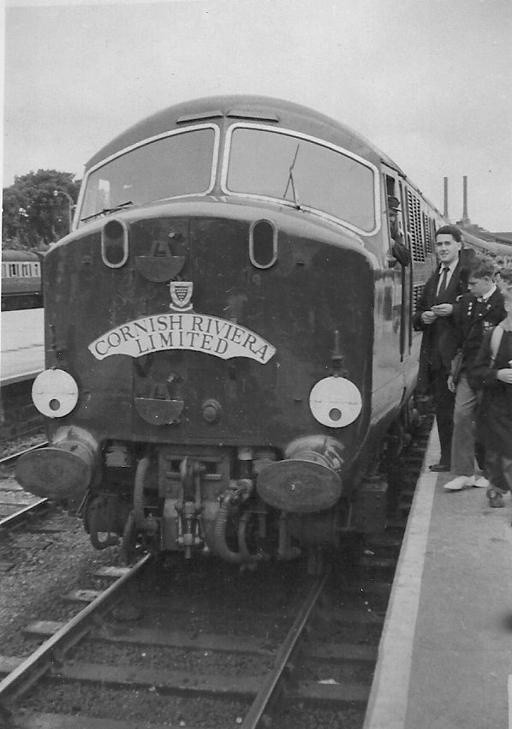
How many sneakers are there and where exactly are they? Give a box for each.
[430,463,505,508]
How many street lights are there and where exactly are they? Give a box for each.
[51,189,76,235]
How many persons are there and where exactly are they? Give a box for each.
[464,265,512,507]
[442,254,505,492]
[477,248,512,294]
[411,225,475,473]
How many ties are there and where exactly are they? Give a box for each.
[438,267,450,296]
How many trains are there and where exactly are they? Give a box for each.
[1,249,46,313]
[10,86,512,588]
